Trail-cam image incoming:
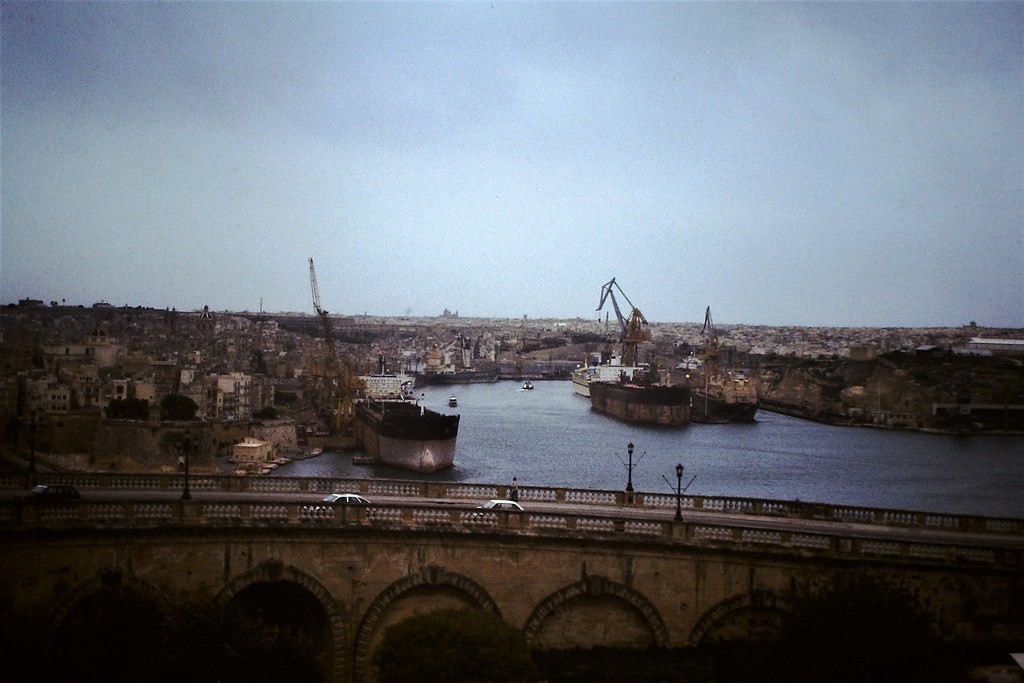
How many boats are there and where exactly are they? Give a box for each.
[448,394,458,407]
[425,332,500,384]
[523,381,534,389]
[353,395,460,476]
[571,351,761,429]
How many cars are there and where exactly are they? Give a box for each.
[299,493,377,518]
[9,484,84,505]
[472,499,525,518]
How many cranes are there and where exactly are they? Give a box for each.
[596,277,652,367]
[298,257,371,431]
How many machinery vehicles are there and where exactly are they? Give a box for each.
[698,305,720,360]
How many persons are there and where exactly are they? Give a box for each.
[510,477,519,502]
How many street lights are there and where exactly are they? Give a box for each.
[25,408,37,473]
[180,426,192,501]
[673,463,684,521]
[625,443,635,491]
